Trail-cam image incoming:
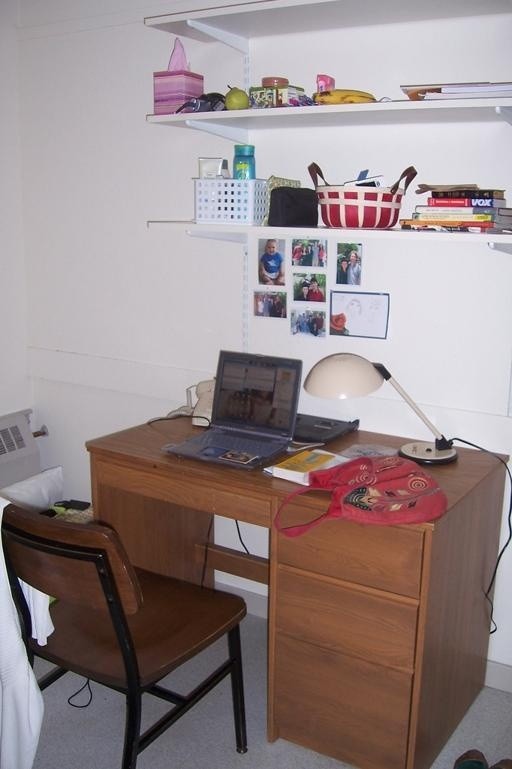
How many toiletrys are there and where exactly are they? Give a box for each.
[221,159,231,179]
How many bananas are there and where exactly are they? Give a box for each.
[312,89,377,104]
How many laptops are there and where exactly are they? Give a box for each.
[165,348,304,472]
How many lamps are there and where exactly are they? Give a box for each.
[303,352,459,465]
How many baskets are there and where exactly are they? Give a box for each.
[306,163,418,229]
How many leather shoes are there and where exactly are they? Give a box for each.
[453,748,512,769]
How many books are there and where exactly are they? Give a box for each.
[398,82,512,100]
[272,449,335,484]
[399,184,511,234]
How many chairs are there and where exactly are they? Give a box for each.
[2,503,249,769]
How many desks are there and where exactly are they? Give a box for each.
[85,416,507,767]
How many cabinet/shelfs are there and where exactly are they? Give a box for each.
[143,1,512,252]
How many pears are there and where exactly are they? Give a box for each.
[224,85,249,110]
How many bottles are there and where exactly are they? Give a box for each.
[232,144,256,180]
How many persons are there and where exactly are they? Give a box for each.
[255,240,360,336]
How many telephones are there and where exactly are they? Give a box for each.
[191,380,218,427]
